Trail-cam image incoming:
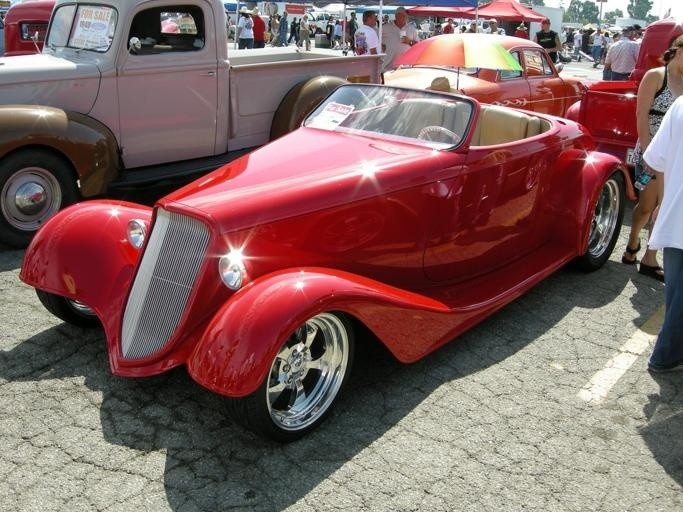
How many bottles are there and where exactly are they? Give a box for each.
[634,167,653,190]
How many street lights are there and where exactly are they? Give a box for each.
[597,0,607,19]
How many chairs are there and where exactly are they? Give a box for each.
[393,96,456,144]
[455,104,540,144]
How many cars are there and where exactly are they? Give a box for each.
[381,31,591,124]
[300,11,339,38]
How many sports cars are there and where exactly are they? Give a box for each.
[18,83,640,446]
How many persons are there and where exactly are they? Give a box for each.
[416,155,468,249]
[622,34,683,282]
[643,96,683,374]
[462,160,504,228]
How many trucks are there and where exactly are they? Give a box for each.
[257,0,315,30]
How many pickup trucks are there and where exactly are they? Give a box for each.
[1,0,393,250]
[4,1,58,57]
[563,18,683,193]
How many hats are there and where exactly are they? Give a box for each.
[488,18,498,23]
[633,24,645,31]
[238,6,253,14]
[622,26,634,32]
[516,23,528,31]
[540,18,551,25]
[251,7,261,16]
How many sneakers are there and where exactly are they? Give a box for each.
[577,54,582,63]
[592,59,605,69]
[646,361,683,374]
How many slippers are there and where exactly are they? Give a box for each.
[638,260,666,283]
[621,238,641,265]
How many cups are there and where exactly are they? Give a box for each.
[399,31,406,43]
